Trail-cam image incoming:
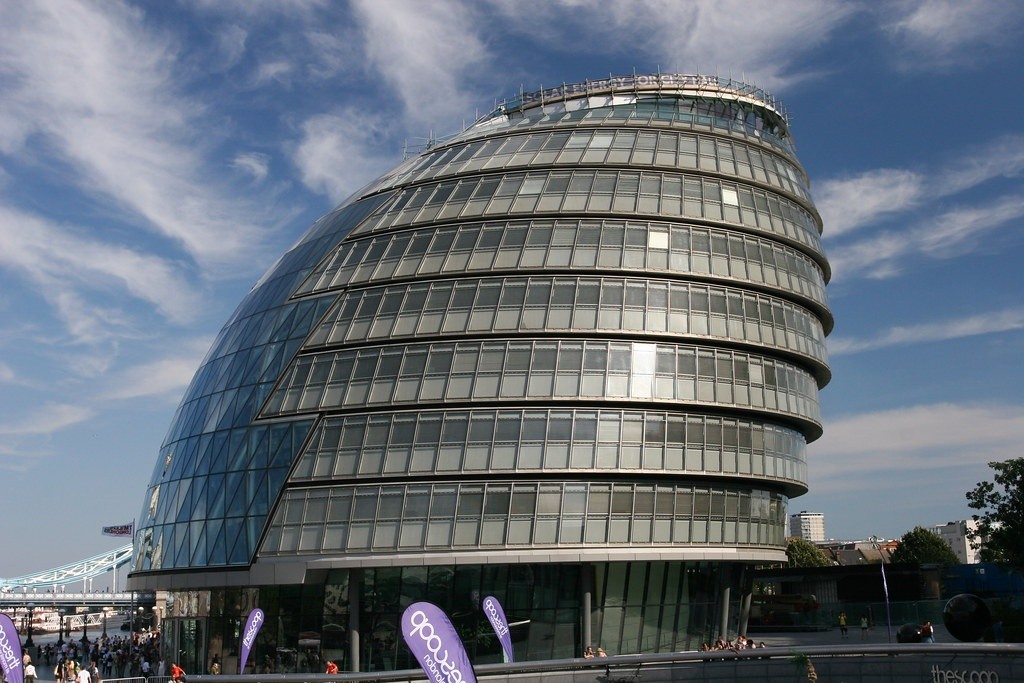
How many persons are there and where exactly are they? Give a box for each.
[212,654,219,667]
[249,649,325,673]
[860,615,868,640]
[920,621,934,643]
[326,661,338,674]
[343,632,408,671]
[0,625,186,683]
[211,663,221,675]
[703,635,766,655]
[583,646,607,660]
[838,611,848,639]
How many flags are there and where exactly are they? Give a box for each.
[102,522,133,538]
